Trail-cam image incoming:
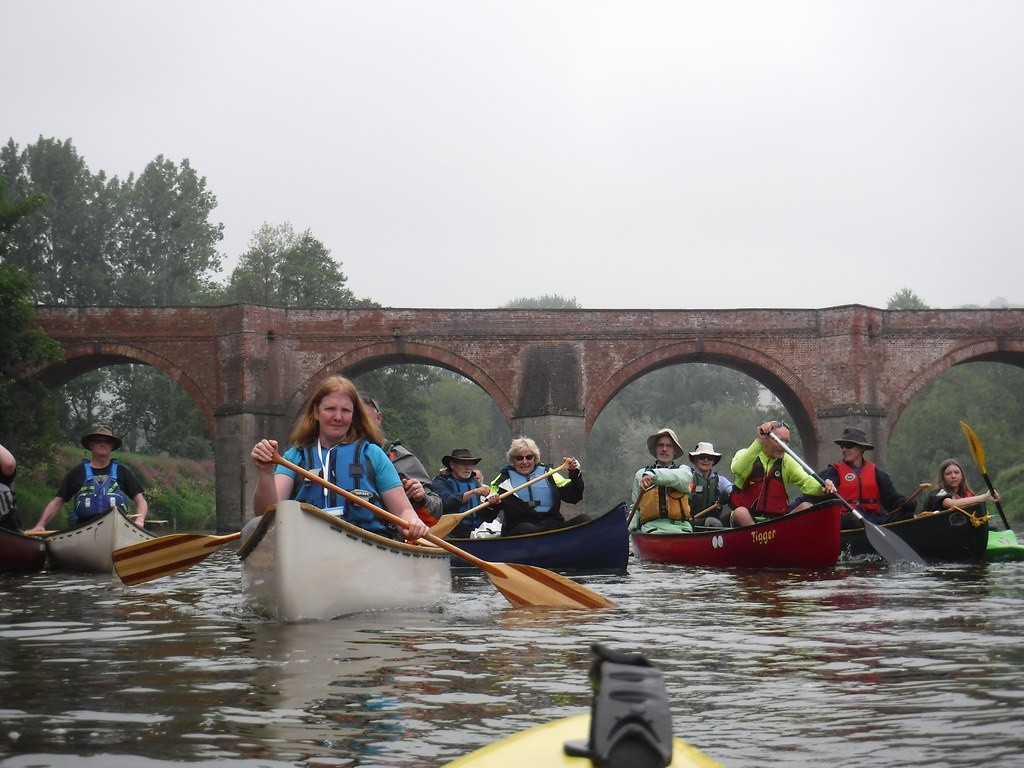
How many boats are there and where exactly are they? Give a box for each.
[984,528,1023,562]
[631,499,848,569]
[235,499,452,623]
[842,498,989,563]
[46,505,158,577]
[446,502,631,575]
[0,527,49,571]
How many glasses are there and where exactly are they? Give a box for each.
[362,395,380,412]
[90,437,112,444]
[838,441,857,449]
[699,455,713,461]
[772,421,790,429]
[515,455,534,461]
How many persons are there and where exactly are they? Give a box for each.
[358,388,443,528]
[790,427,919,529]
[729,420,838,528]
[627,429,693,533]
[482,435,592,536]
[28,426,148,528]
[429,446,489,539]
[252,374,429,543]
[0,443,20,533]
[688,441,755,525]
[922,458,1000,512]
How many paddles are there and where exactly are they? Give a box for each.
[426,456,578,539]
[758,426,929,568]
[885,483,934,517]
[22,530,60,536]
[686,501,722,522]
[958,420,1011,531]
[110,480,414,588]
[260,446,621,611]
[626,478,653,529]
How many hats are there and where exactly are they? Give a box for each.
[834,427,875,451]
[689,442,722,466]
[442,449,482,469]
[647,428,684,459]
[79,427,124,451]
